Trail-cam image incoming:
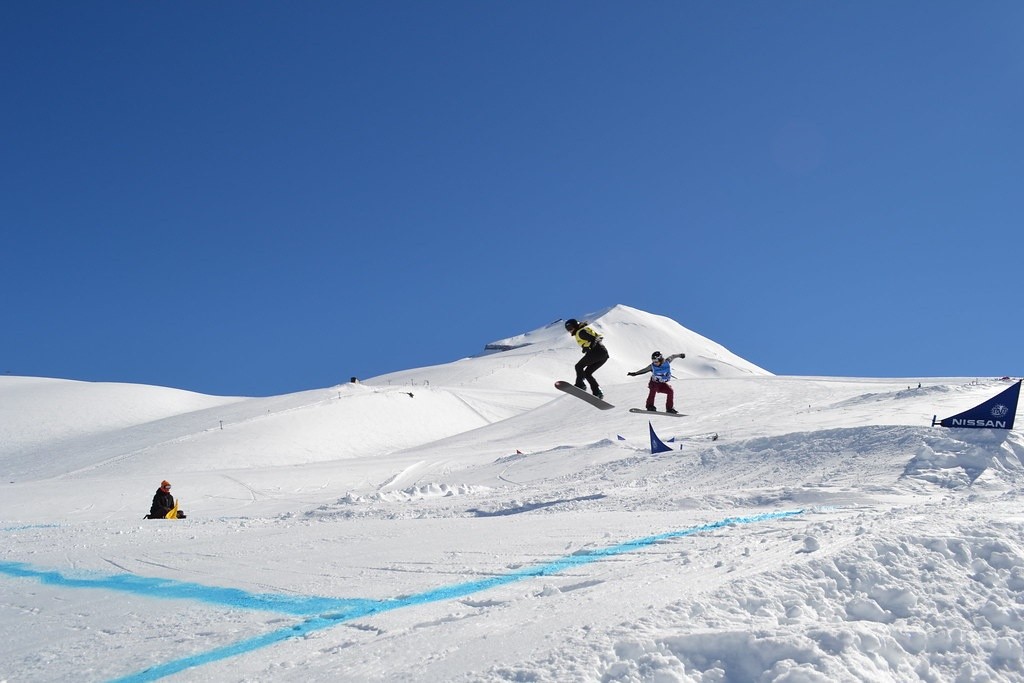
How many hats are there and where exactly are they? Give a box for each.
[161,480,171,489]
[652,352,662,361]
[565,319,577,329]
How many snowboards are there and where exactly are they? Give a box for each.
[629,407,690,418]
[554,380,615,410]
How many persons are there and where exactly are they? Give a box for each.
[565,316,608,399]
[629,348,686,414]
[148,478,185,518]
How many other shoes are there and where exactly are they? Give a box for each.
[666,409,678,414]
[647,406,656,411]
[595,394,603,399]
[574,384,586,390]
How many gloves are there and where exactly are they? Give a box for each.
[627,373,635,376]
[680,353,685,358]
